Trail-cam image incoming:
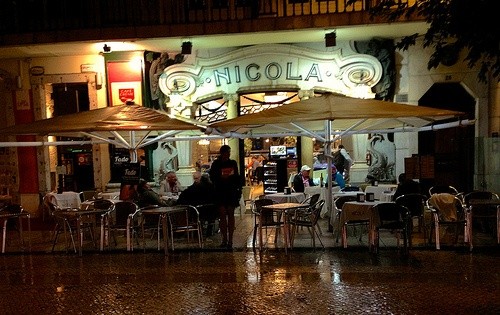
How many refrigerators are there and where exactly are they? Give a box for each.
[262,159,288,194]
[281,156,299,192]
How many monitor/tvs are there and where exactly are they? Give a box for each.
[269,145,287,156]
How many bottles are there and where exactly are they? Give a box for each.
[374,181,378,187]
[320,173,323,188]
[266,170,276,174]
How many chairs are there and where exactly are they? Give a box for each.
[1,186,500,256]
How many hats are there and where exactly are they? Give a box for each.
[331,165,336,169]
[137,179,148,192]
[301,165,311,171]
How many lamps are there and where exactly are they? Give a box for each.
[324,27,336,47]
[16,76,22,88]
[103,43,111,53]
[182,36,192,55]
[96,72,102,86]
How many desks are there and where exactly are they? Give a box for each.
[0,211,31,255]
[137,206,188,267]
[365,184,397,200]
[264,192,305,205]
[260,203,310,251]
[320,190,364,222]
[64,207,110,257]
[339,201,402,248]
[304,184,341,215]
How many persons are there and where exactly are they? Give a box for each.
[392,173,418,201]
[210,145,238,246]
[294,165,316,193]
[333,144,353,173]
[247,156,256,169]
[149,53,169,112]
[197,174,214,225]
[252,156,264,171]
[160,172,184,197]
[178,172,202,205]
[326,165,345,189]
[139,181,165,227]
[366,40,392,99]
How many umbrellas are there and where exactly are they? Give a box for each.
[0,98,205,163]
[204,92,464,229]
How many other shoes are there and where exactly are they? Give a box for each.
[221,240,227,247]
[228,242,232,248]
